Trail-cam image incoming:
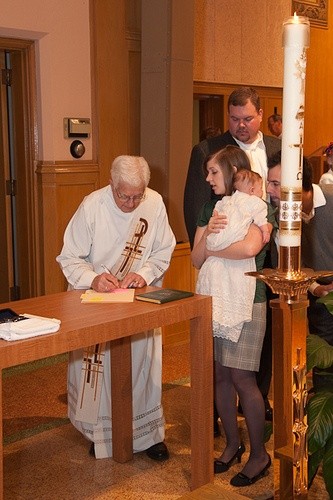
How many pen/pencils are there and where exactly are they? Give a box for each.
[100,264,120,288]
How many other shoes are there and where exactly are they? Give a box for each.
[237,400,273,421]
[213,416,221,436]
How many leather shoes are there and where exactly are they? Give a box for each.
[146,442,169,461]
[90,442,95,455]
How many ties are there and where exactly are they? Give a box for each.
[245,148,255,172]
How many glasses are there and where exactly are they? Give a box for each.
[115,183,146,202]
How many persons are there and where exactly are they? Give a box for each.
[191,145,275,486]
[56,156,176,459]
[184,88,283,436]
[207,168,270,251]
[319,144,333,184]
[267,151,333,390]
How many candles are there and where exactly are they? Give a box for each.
[277,12,311,278]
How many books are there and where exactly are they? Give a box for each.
[81,288,135,303]
[136,289,191,304]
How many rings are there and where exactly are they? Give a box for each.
[135,281,138,283]
[105,287,107,290]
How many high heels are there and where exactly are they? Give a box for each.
[214,441,246,473]
[230,453,272,487]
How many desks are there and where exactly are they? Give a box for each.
[0,285,214,491]
